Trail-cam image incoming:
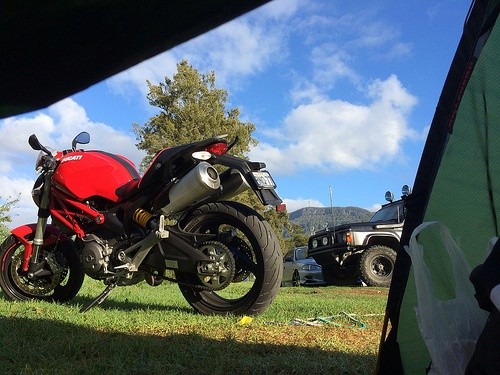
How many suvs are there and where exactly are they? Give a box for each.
[305,185,413,288]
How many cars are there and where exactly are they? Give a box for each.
[280,247,330,287]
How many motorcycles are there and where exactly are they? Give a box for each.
[0,132,286,321]
